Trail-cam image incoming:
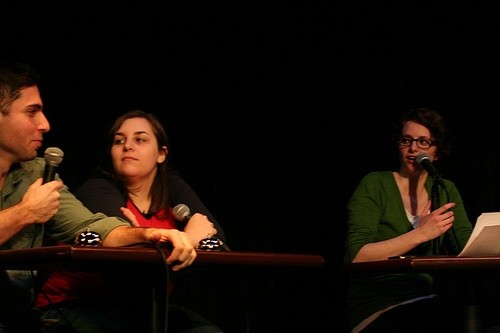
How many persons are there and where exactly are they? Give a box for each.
[344,106,474,333]
[0,67,197,333]
[36,110,232,333]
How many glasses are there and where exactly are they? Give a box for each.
[397,137,438,150]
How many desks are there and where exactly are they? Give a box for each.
[0,245,323,333]
[344,256,500,333]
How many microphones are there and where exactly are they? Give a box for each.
[415,151,446,189]
[171,203,231,251]
[35,146,64,231]
